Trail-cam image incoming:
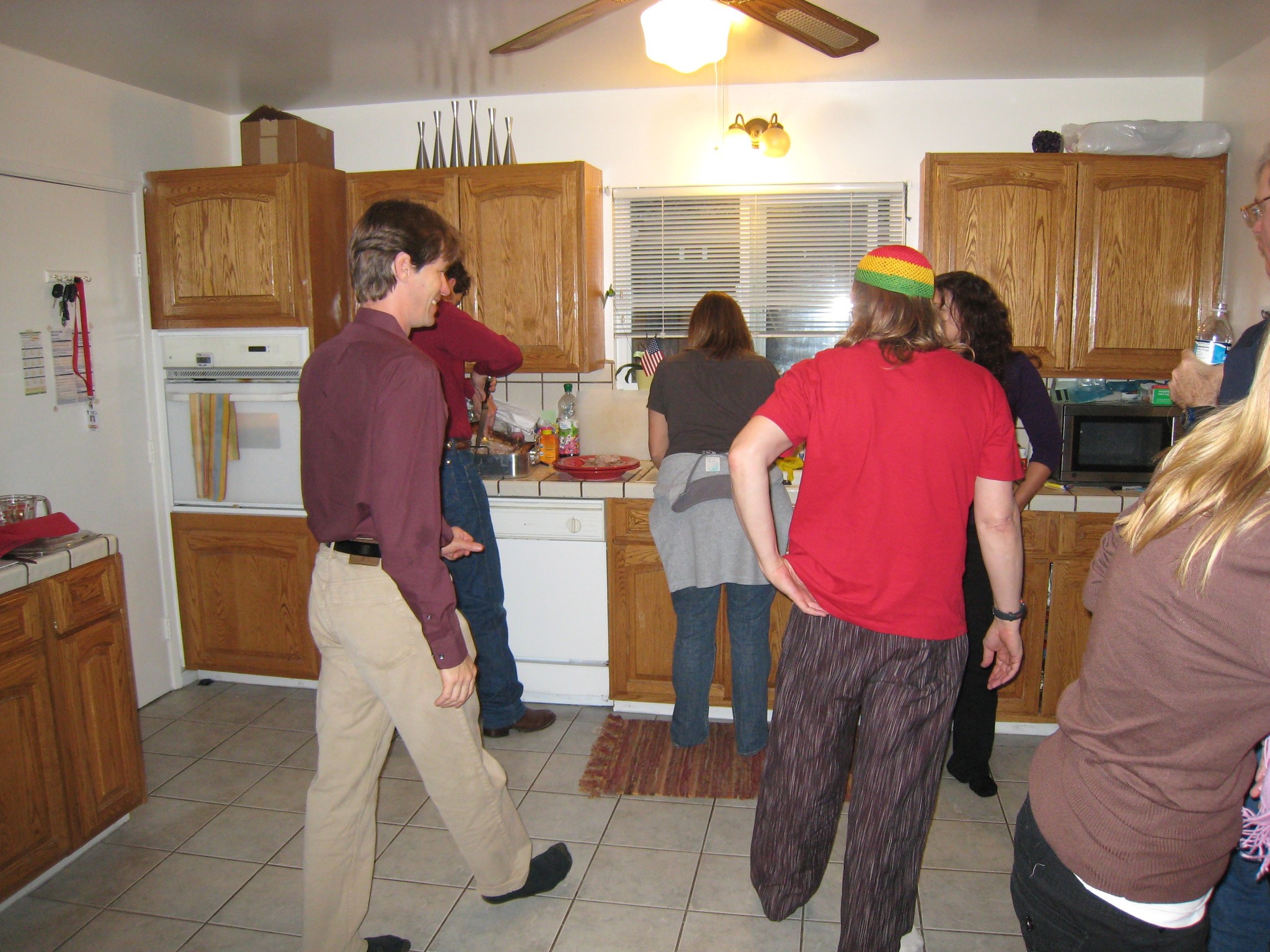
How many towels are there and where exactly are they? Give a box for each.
[189,392,240,502]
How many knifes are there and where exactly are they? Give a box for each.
[475,376,493,448]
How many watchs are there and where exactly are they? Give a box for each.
[992,598,1027,621]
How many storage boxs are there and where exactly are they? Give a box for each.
[539,433,561,465]
[1149,388,1174,406]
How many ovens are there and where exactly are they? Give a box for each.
[151,325,312,520]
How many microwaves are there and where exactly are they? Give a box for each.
[1050,400,1185,487]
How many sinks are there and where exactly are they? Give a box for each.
[780,470,802,487]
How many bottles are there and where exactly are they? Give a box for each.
[468,99,483,167]
[503,117,518,165]
[486,108,501,165]
[558,383,580,457]
[537,426,559,467]
[450,101,464,168]
[432,111,447,168]
[416,121,430,169]
[1192,303,1234,366]
[511,427,524,441]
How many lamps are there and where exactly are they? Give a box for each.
[723,110,791,161]
[641,0,730,74]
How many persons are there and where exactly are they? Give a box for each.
[406,262,557,741]
[1008,141,1270,952]
[296,201,575,952]
[647,292,782,753]
[729,246,1025,951]
[932,269,1060,796]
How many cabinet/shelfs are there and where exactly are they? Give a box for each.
[604,496,794,705]
[145,167,306,327]
[925,150,1227,375]
[348,162,581,371]
[170,509,321,681]
[994,514,1117,724]
[0,558,148,903]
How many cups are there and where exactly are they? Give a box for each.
[635,369,654,390]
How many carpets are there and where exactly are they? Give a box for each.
[578,717,855,802]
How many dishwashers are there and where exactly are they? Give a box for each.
[487,498,610,703]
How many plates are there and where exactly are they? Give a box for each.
[552,454,641,480]
[1122,394,1138,400]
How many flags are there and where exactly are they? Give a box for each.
[640,338,665,377]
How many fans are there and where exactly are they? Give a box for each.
[489,0,881,57]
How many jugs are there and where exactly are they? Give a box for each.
[0,494,51,526]
